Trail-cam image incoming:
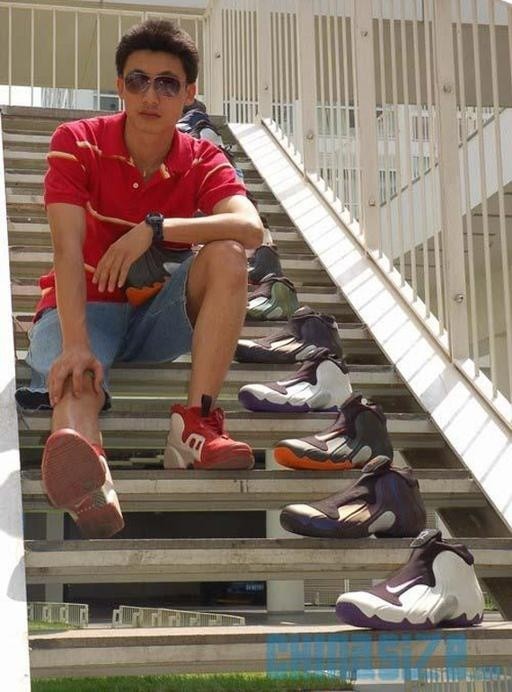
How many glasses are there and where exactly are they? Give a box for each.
[123,72,182,98]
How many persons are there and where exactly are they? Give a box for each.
[12,18,264,539]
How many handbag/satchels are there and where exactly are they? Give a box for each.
[124,241,196,305]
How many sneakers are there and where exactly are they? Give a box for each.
[164,394,254,471]
[176,94,299,319]
[336,527,484,629]
[43,429,124,538]
[236,306,344,364]
[280,456,426,537]
[274,393,392,471]
[237,347,354,413]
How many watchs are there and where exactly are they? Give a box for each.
[145,211,163,246]
